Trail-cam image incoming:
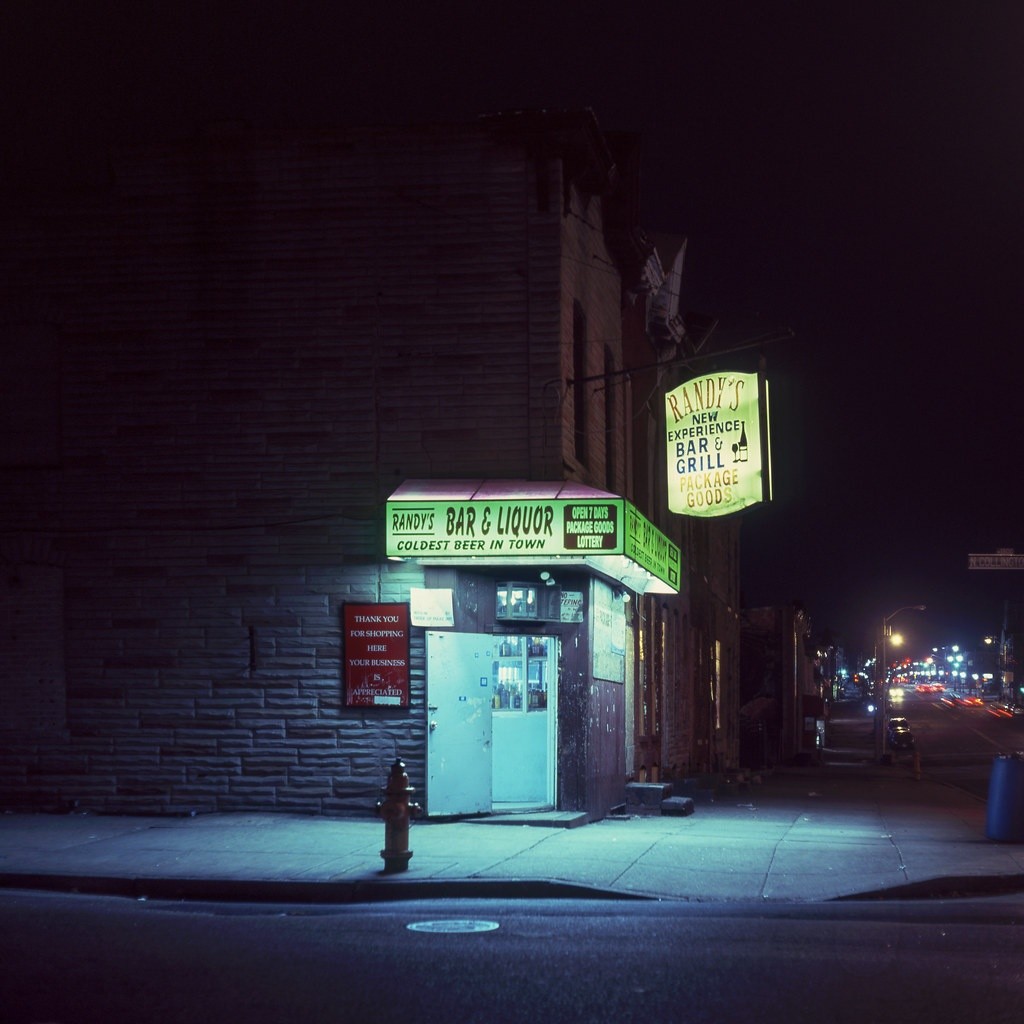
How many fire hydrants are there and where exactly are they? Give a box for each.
[375,757,422,875]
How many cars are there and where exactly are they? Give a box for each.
[909,679,926,684]
[1004,702,1024,716]
[916,683,944,694]
[854,673,870,686]
[887,716,915,752]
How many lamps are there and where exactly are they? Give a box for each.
[683,311,719,354]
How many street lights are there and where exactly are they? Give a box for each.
[984,637,1008,701]
[896,661,945,683]
[874,635,902,685]
[882,605,926,754]
[947,645,979,696]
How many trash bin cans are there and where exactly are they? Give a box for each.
[985,752,1024,843]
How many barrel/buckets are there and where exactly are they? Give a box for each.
[984,750,1024,843]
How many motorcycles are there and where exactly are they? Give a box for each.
[867,688,876,700]
